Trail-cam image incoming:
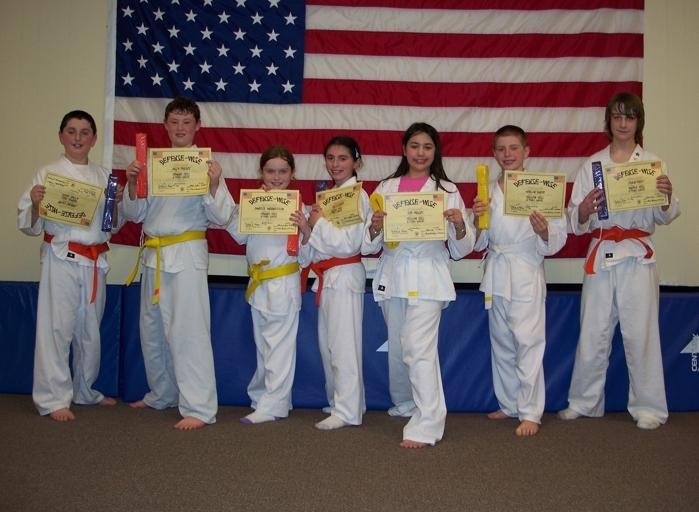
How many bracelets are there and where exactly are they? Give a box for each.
[371,226,378,234]
[456,224,466,233]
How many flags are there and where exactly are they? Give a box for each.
[98,1,645,259]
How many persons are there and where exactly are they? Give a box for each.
[360,121,476,448]
[472,125,567,438]
[559,92,679,430]
[123,98,236,431]
[305,133,372,431]
[18,110,124,421]
[225,145,315,425]
